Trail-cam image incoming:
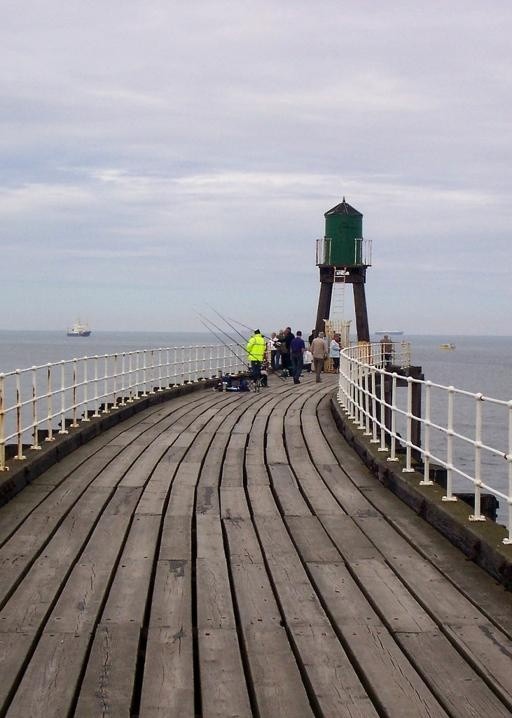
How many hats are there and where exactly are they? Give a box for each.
[319,332,324,337]
[297,331,302,336]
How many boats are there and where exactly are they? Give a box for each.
[374,329,404,335]
[440,342,457,350]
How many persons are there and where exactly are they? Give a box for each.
[246,328,266,375]
[379,334,392,368]
[268,327,344,384]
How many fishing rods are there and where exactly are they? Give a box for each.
[194,306,287,382]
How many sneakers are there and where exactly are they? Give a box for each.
[294,379,300,384]
[332,368,337,374]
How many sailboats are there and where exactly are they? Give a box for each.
[67,317,91,337]
